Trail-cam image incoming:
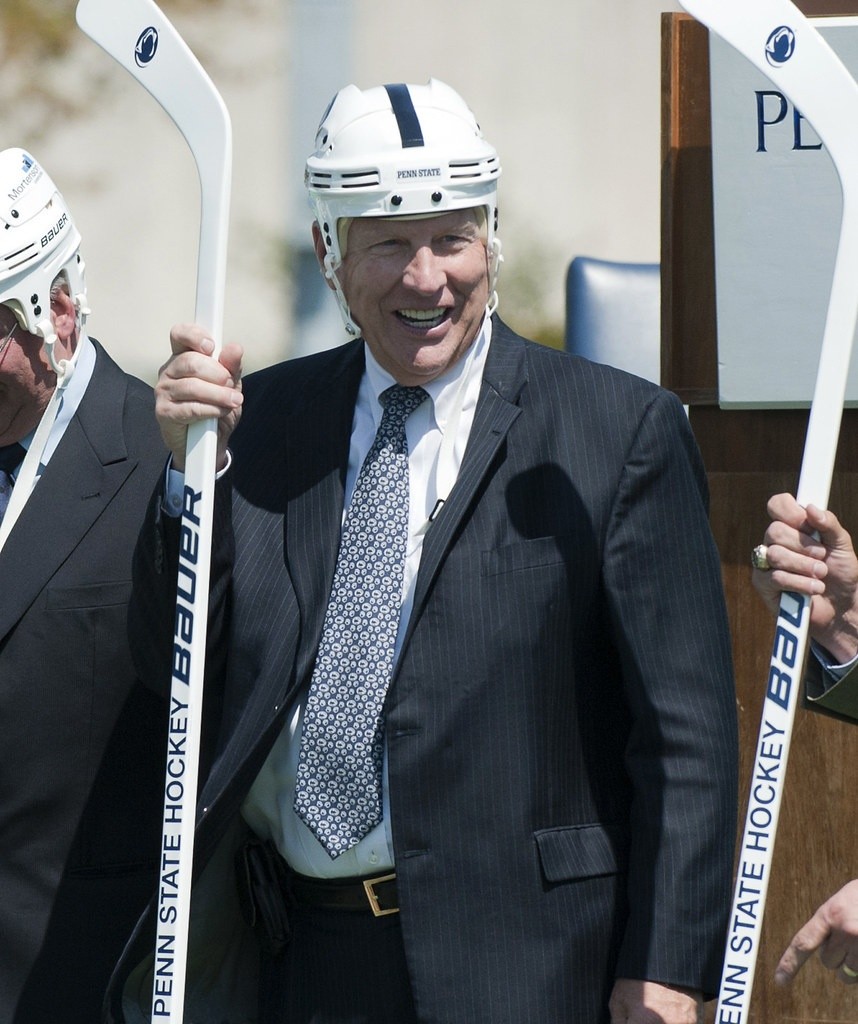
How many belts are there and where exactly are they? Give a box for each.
[274,872,402,918]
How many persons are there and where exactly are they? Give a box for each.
[1,148,176,1024]
[155,83,738,1024]
[751,493,858,1024]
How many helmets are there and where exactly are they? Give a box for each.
[0,144,89,346]
[303,76,506,279]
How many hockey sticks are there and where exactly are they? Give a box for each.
[68,0,237,1024]
[669,1,858,1023]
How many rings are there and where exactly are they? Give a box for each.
[753,544,769,568]
[841,963,857,977]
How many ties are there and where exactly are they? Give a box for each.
[292,385,433,861]
[0,438,28,534]
